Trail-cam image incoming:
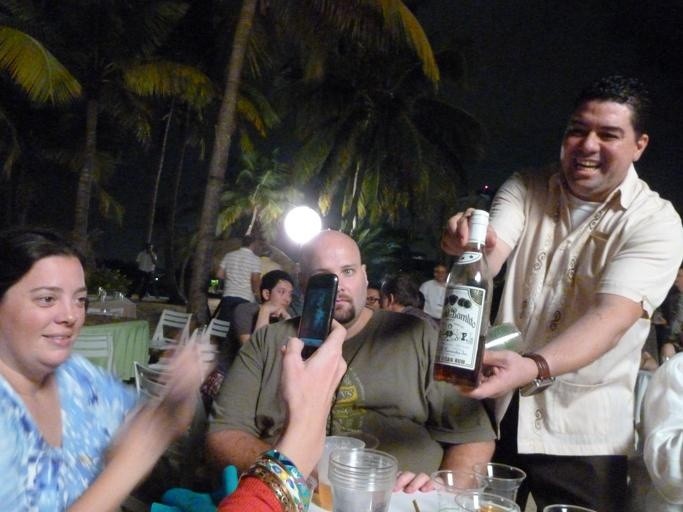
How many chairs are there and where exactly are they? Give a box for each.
[75,308,232,434]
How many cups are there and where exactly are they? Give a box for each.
[484,323,525,372]
[430,462,527,511]
[317,436,399,511]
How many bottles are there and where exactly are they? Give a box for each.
[434,210,494,389]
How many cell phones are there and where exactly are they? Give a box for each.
[297,272,340,359]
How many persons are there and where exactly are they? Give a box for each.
[125,242,158,303]
[199,225,497,493]
[0,223,216,511]
[434,70,683,510]
[200,234,681,511]
